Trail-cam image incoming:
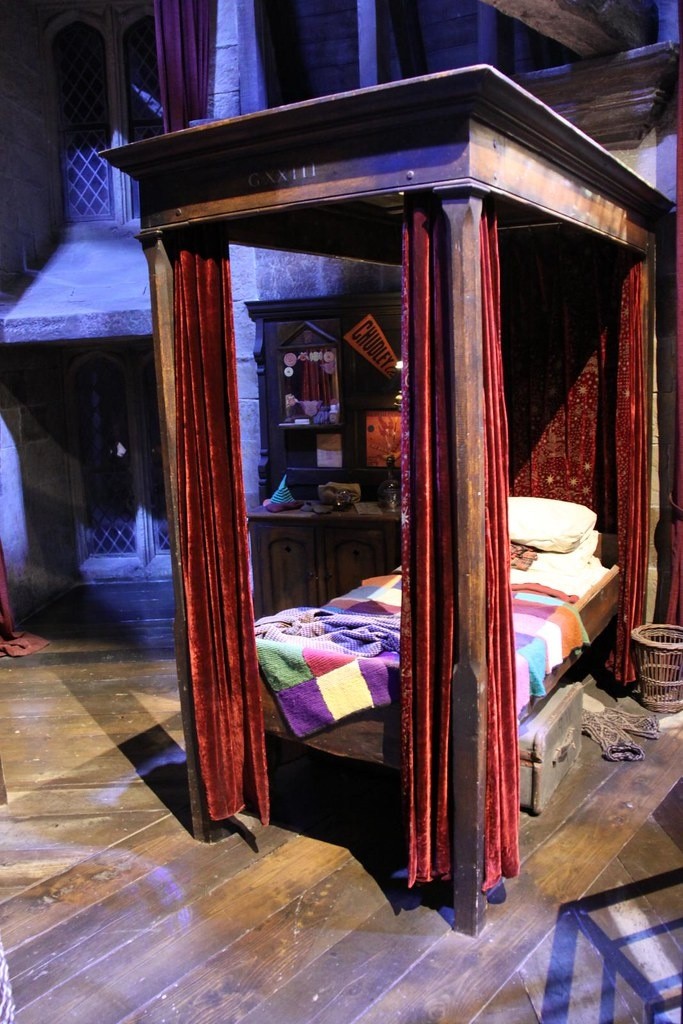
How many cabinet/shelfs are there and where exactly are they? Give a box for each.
[244,288,406,498]
[243,504,401,619]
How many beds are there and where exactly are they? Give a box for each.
[92,63,676,934]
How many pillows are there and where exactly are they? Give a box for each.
[505,495,599,552]
[532,530,600,567]
